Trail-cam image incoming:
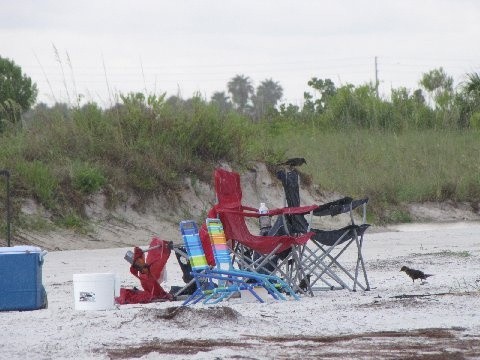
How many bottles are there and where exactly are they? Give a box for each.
[259,203,269,232]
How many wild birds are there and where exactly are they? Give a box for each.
[399,265,434,282]
[295,274,313,294]
[277,158,307,169]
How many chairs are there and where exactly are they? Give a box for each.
[180,220,277,304]
[206,218,298,300]
[214,167,318,297]
[276,167,371,292]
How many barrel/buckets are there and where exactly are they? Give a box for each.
[72,272,121,310]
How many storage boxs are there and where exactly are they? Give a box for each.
[0,245,48,311]
[72,273,115,311]
